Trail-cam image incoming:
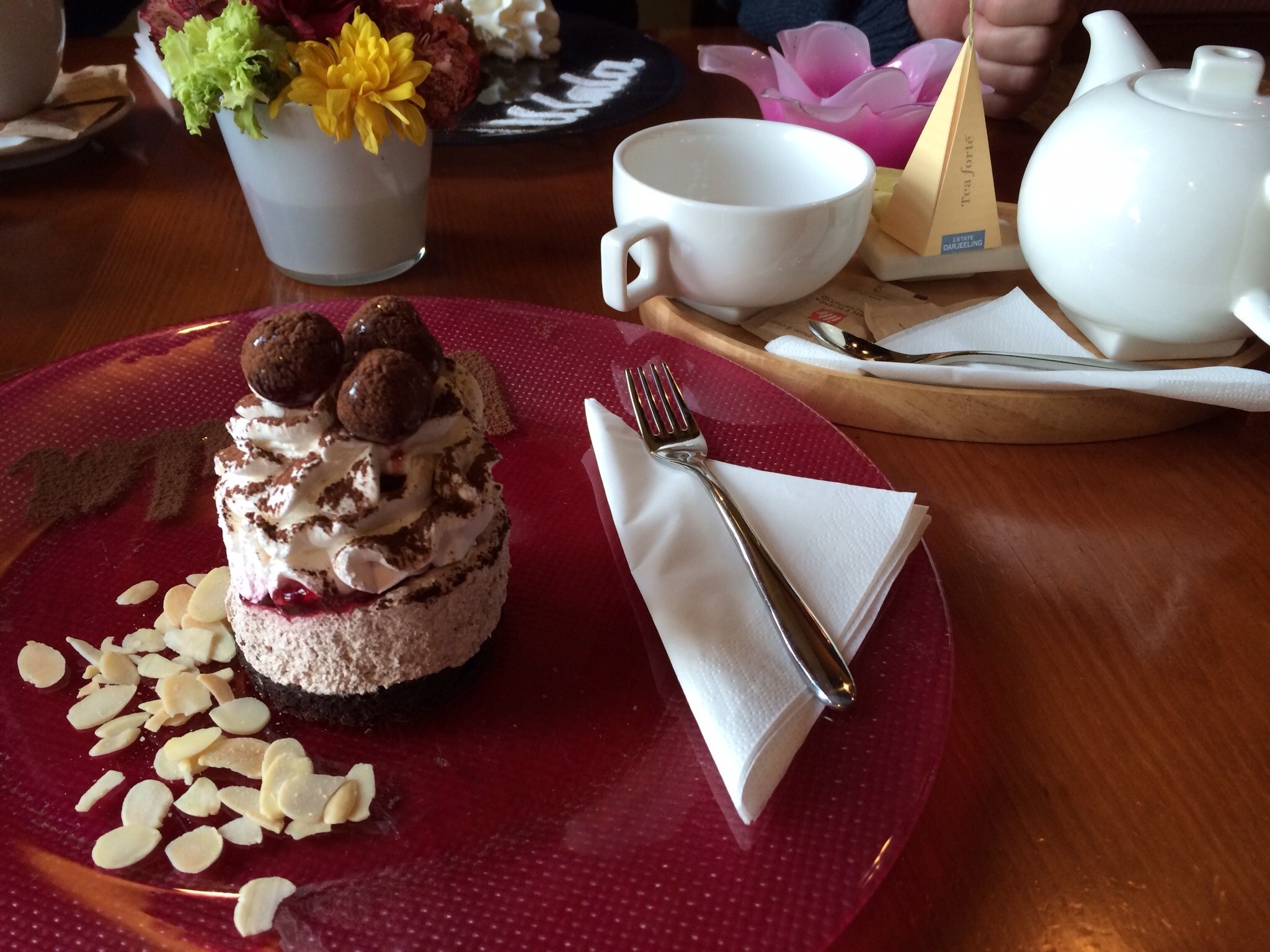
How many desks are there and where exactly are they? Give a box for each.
[1,33,1266,951]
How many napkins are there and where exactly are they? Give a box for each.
[583,395,928,826]
[764,286,1270,416]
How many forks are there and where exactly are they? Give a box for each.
[621,361,858,711]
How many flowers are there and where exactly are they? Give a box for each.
[137,0,484,156]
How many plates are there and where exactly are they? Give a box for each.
[639,198,1206,442]
[0,90,136,168]
[0,297,956,952]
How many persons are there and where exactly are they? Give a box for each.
[733,0,1077,119]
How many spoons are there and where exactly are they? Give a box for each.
[806,319,1138,371]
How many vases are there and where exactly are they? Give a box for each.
[212,92,434,288]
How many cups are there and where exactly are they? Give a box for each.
[1,0,64,119]
[599,117,877,323]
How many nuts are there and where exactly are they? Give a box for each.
[18,566,377,936]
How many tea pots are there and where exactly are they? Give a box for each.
[1014,9,1270,362]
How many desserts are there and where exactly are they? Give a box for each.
[214,295,512,728]
